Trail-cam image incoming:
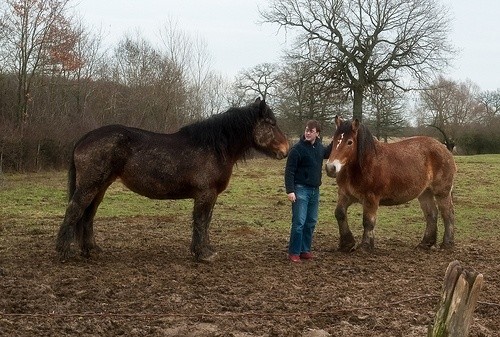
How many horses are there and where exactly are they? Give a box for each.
[57,96,290,265]
[325,114,456,256]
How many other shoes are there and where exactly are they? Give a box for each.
[288,254,301,263]
[300,252,317,260]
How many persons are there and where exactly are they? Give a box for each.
[285,121,333,263]
